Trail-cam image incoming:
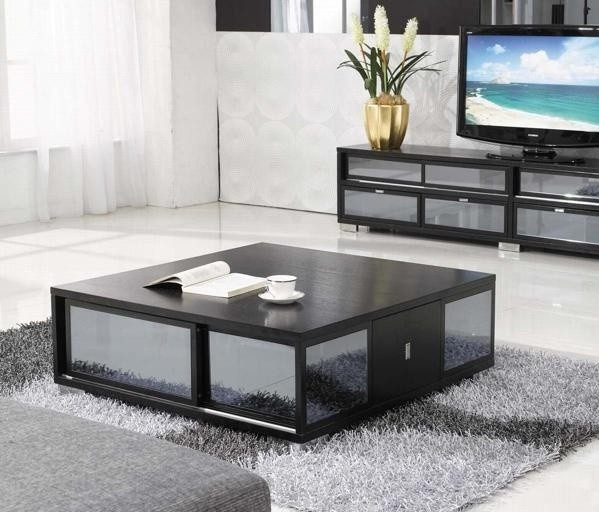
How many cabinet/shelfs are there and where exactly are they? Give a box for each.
[336,142,597,261]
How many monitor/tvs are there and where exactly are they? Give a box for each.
[456,25,599,164]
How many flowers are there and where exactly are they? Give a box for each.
[334,0,449,102]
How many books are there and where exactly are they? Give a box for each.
[142,260,267,298]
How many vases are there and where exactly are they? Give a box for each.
[365,100,410,152]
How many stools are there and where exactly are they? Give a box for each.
[0,398,272,512]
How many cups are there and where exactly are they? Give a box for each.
[267,276,296,296]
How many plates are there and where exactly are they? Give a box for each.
[258,292,304,305]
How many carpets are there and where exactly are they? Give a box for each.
[2,316,597,510]
